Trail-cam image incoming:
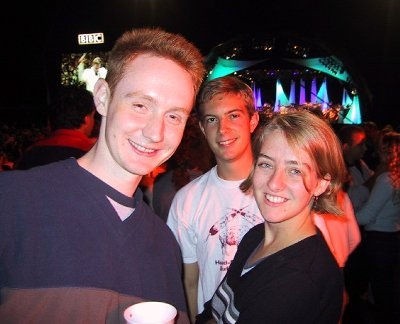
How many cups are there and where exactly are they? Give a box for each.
[124,302,177,324]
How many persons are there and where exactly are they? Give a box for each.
[166,75,333,324]
[339,124,400,304]
[14,88,97,171]
[193,108,350,324]
[0,29,207,324]
[153,116,217,222]
